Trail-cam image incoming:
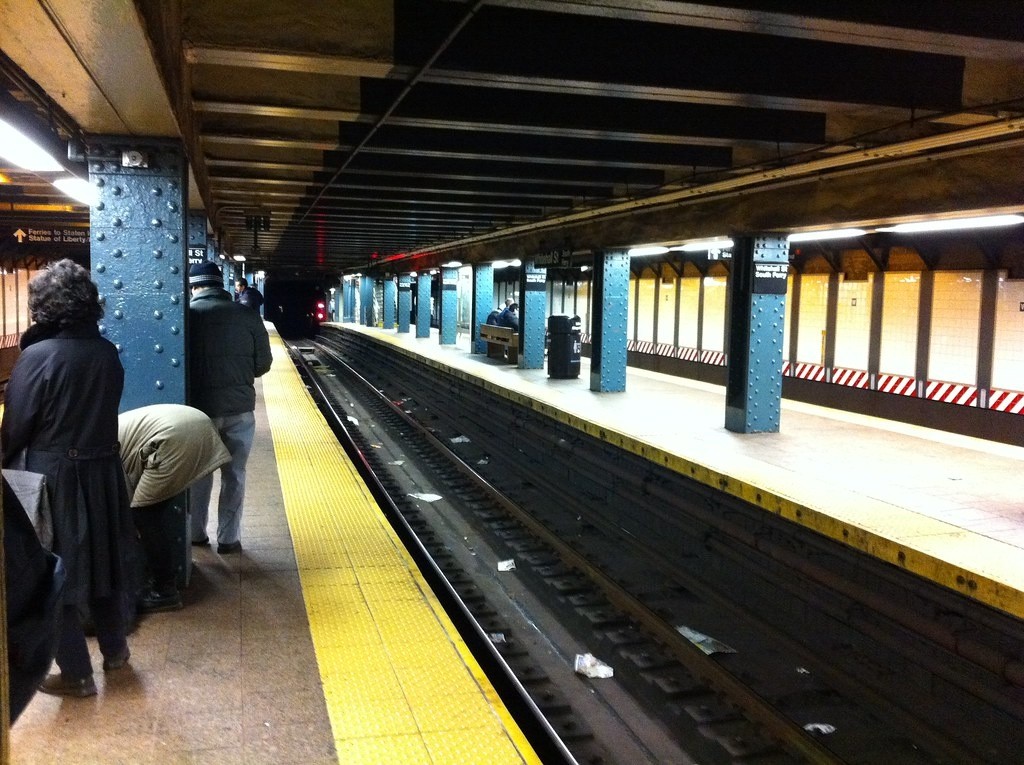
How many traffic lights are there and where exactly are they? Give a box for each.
[318,302,325,320]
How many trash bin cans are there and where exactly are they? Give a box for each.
[547,314,582,380]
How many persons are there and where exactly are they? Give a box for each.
[486,298,519,333]
[190,262,272,553]
[235,278,263,316]
[0,259,130,728]
[118,404,232,612]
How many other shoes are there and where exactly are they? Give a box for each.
[134,579,187,613]
[191,536,209,546]
[217,540,242,554]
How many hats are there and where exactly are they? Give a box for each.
[188,259,224,288]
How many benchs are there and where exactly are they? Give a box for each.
[480,324,519,364]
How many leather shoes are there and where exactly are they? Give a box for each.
[102,643,131,670]
[37,670,98,698]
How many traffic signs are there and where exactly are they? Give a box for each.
[10,228,90,245]
